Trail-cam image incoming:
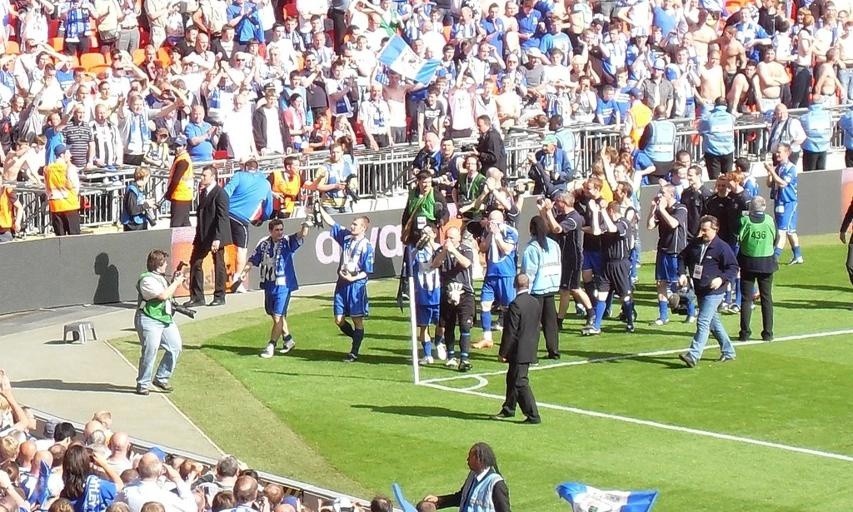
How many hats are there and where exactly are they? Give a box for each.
[540,135,557,146]
[651,57,665,71]
[525,46,542,57]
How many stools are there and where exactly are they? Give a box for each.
[62,320,99,345]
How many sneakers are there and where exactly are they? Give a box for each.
[417,356,433,365]
[342,353,358,363]
[581,326,601,336]
[681,315,697,324]
[135,384,150,397]
[153,378,173,392]
[626,322,634,333]
[260,337,297,360]
[493,409,517,418]
[720,301,741,315]
[471,338,494,349]
[678,351,696,368]
[521,416,542,424]
[648,317,671,328]
[714,354,736,363]
[434,341,447,360]
[446,357,473,371]
[788,255,804,266]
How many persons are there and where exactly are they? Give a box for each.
[2,1,853,233]
[186,144,853,423]
[1,375,512,512]
[135,248,186,394]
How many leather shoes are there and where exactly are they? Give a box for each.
[182,297,207,307]
[209,299,227,306]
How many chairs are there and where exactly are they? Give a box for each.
[3,37,174,75]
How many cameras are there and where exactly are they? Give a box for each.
[142,207,156,227]
[342,172,361,204]
[170,260,187,284]
[167,298,197,319]
